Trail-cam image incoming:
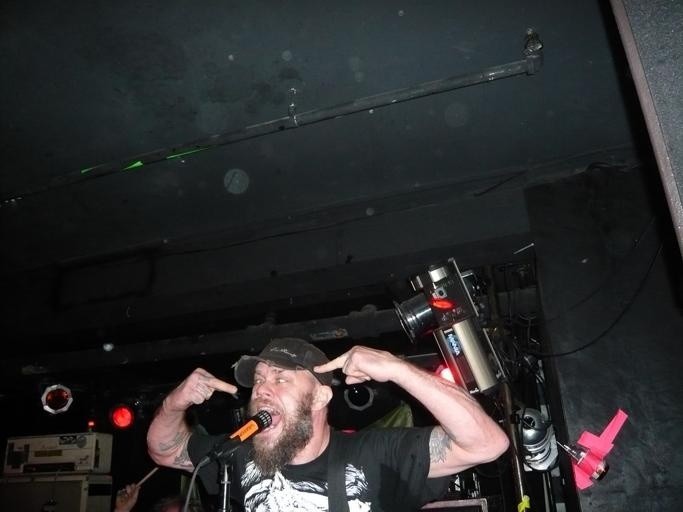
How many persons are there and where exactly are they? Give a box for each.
[143,337,509,511]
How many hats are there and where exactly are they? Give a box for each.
[234,337,333,388]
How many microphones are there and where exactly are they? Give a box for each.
[198,410,276,471]
